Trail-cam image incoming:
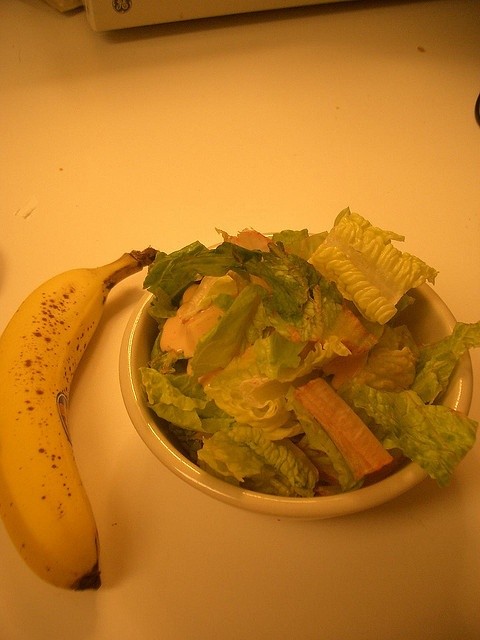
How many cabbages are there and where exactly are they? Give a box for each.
[138,203,480,504]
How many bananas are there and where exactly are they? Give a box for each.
[1,245,158,593]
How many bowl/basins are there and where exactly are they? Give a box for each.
[118,233,473,519]
